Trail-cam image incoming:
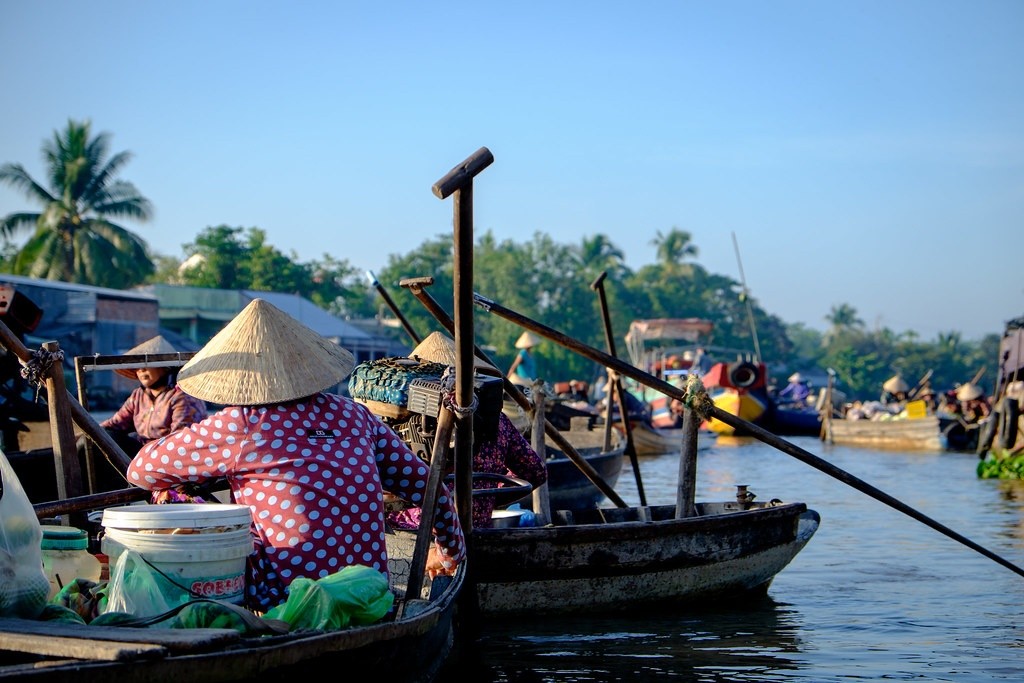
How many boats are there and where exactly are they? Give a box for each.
[507,401,627,512]
[0,442,468,683]
[597,318,768,433]
[822,404,969,452]
[413,482,821,619]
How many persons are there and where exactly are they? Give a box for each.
[921,387,938,410]
[594,377,607,401]
[781,372,809,406]
[75,334,209,453]
[515,330,541,380]
[503,375,532,414]
[126,298,467,613]
[880,376,909,403]
[958,383,991,419]
[409,332,533,529]
[692,345,712,375]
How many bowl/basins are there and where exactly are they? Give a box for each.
[491,509,524,528]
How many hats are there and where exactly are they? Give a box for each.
[114,335,188,379]
[177,298,356,406]
[957,383,981,400]
[516,331,542,348]
[884,376,906,393]
[789,372,807,385]
[409,332,504,376]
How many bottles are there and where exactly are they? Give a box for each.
[40,526,101,605]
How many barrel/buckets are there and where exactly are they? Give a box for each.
[101,503,254,629]
